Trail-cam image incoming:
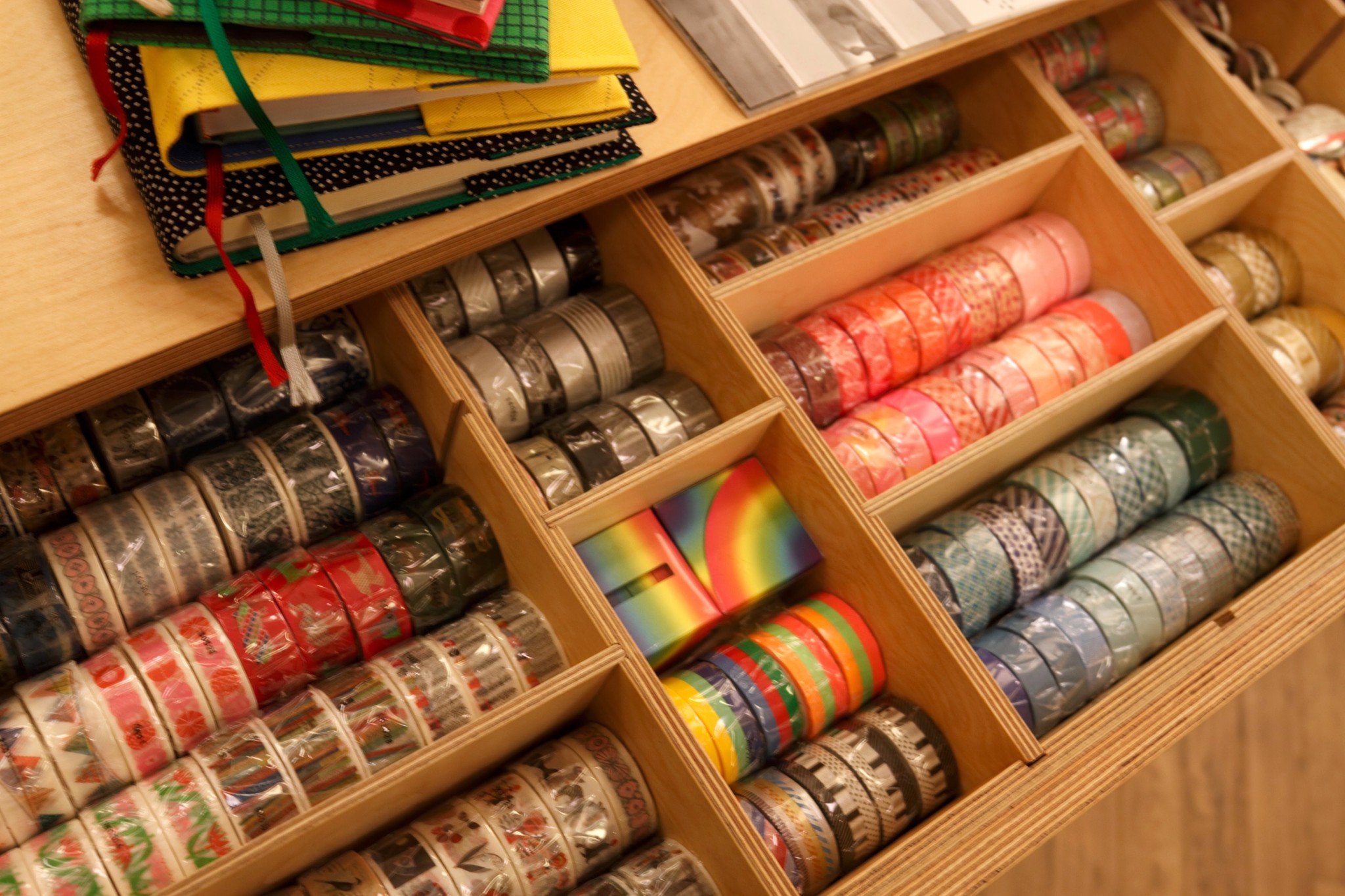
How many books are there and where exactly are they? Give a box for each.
[60,0,658,279]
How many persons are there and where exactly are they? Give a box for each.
[828,6,895,65]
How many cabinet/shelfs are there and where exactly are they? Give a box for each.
[0,0,1344,895]
